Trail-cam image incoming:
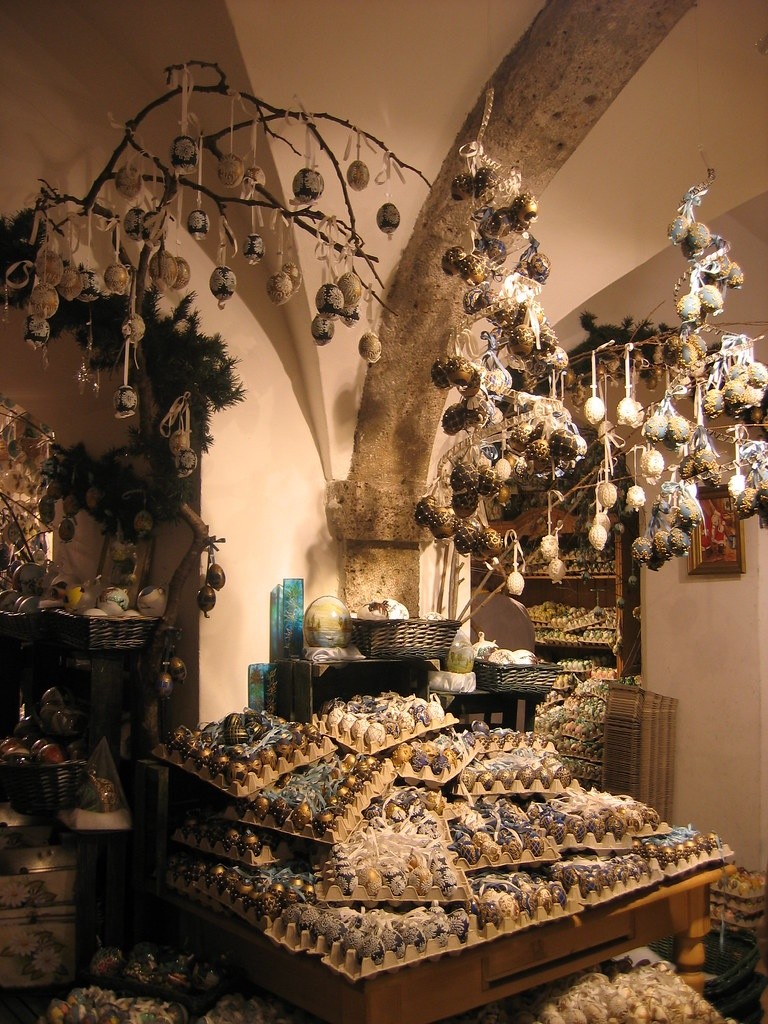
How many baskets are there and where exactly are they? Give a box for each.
[471,658,565,695]
[3,759,91,816]
[350,615,464,660]
[647,927,765,1023]
[3,607,163,650]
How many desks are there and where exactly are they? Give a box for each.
[131,864,738,1024]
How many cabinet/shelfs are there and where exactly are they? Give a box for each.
[469,507,640,680]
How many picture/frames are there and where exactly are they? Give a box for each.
[686,483,746,575]
